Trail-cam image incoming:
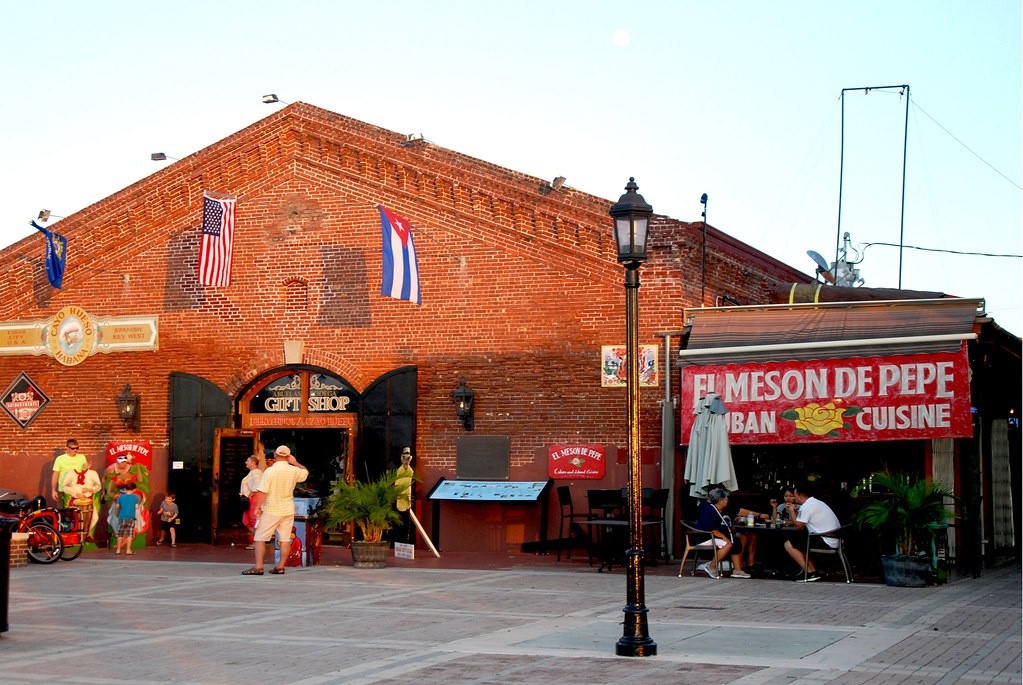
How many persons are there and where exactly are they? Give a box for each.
[770,488,802,527]
[157,492,179,547]
[694,488,769,578]
[52,438,87,508]
[115,482,140,555]
[784,485,841,582]
[240,446,309,575]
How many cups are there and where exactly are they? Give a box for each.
[741,517,745,521]
[284,339,303,364]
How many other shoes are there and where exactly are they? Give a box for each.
[126,551,135,555]
[796,573,820,582]
[172,544,176,547]
[156,540,162,545]
[115,551,120,554]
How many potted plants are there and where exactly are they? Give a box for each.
[849,473,961,586]
[318,471,417,567]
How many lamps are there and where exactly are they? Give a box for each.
[117,384,140,431]
[453,383,474,431]
[542,177,566,193]
[151,152,181,163]
[38,209,64,221]
[401,133,423,146]
[262,94,292,106]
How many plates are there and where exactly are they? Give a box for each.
[757,524,765,525]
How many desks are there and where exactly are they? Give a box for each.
[728,523,804,574]
[573,518,661,573]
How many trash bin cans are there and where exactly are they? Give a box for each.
[274,488,321,568]
[0,518,19,632]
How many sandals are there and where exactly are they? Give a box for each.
[269,567,284,574]
[242,567,263,575]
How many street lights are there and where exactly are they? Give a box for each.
[609,178,656,657]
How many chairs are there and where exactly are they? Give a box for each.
[804,521,855,584]
[678,520,724,579]
[553,483,669,568]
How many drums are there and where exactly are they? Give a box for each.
[74,498,94,541]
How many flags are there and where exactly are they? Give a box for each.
[379,205,421,305]
[197,196,236,287]
[32,221,67,288]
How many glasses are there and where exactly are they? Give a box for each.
[69,447,78,450]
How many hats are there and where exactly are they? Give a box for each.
[275,445,289,456]
[116,456,127,462]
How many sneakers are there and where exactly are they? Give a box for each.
[732,570,750,578]
[705,564,721,578]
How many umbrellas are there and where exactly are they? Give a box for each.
[684,392,738,500]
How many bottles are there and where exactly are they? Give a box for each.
[775,513,780,527]
[771,516,773,523]
[751,464,771,490]
[747,511,754,527]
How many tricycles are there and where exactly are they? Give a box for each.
[0,490,84,564]
